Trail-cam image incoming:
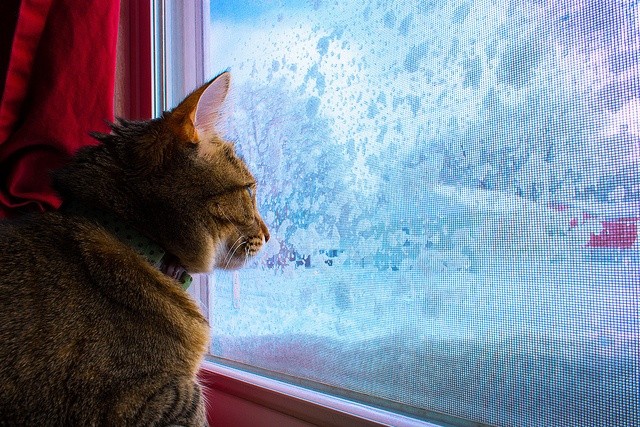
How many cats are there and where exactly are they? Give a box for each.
[0,68,270,427]
